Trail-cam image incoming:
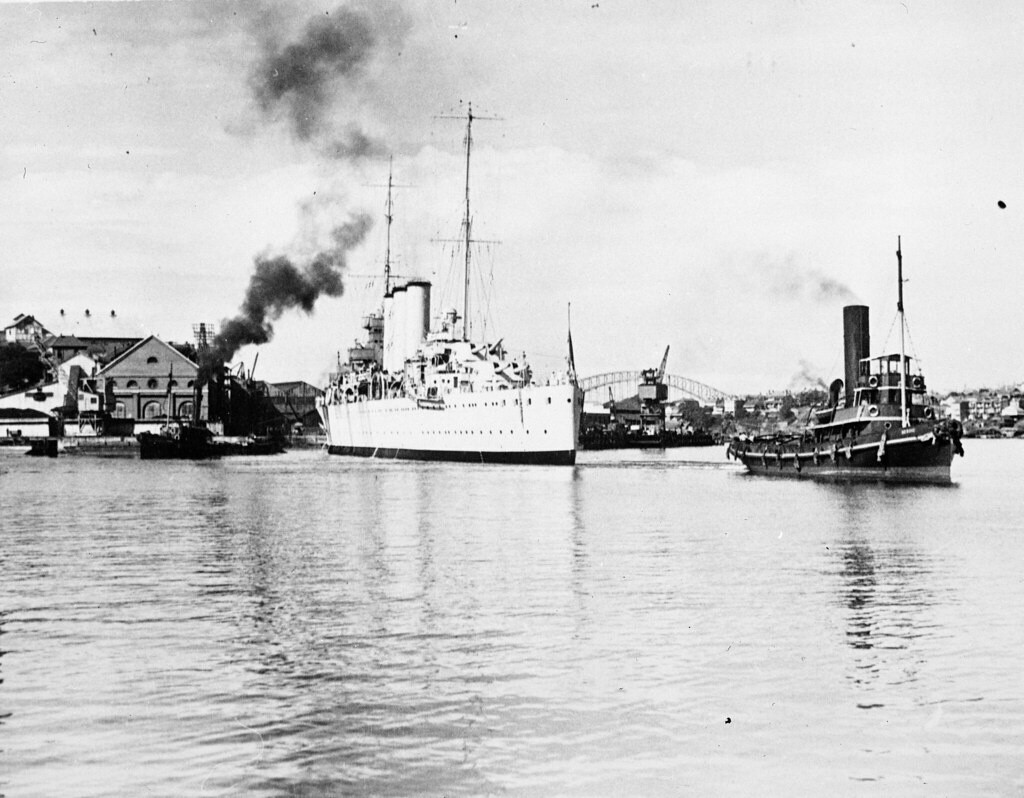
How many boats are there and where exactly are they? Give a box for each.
[136,382,281,459]
[729,235,964,486]
[313,99,585,466]
[31,436,79,454]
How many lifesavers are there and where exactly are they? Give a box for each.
[884,421,892,429]
[869,375,879,387]
[867,404,880,417]
[911,377,921,389]
[924,406,933,420]
